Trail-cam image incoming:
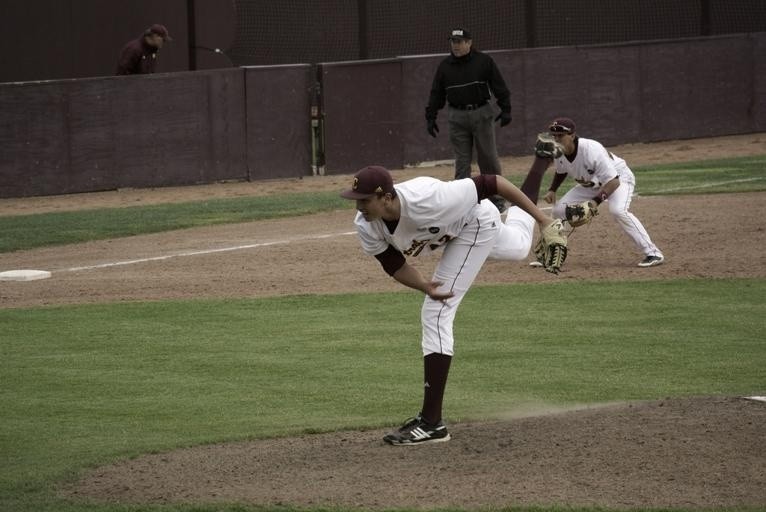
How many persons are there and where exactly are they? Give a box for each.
[425,28,512,211]
[115,24,174,75]
[341,166,568,445]
[529,119,665,267]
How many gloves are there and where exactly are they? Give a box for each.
[427,119,439,138]
[494,110,512,128]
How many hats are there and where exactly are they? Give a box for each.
[150,25,173,42]
[448,28,475,40]
[340,166,393,200]
[548,118,576,134]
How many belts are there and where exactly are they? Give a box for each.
[450,101,487,110]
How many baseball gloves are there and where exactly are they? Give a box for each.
[533,217,567,273]
[565,199,597,227]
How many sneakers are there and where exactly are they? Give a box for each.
[529,261,543,266]
[535,134,566,159]
[638,256,664,267]
[383,412,450,445]
